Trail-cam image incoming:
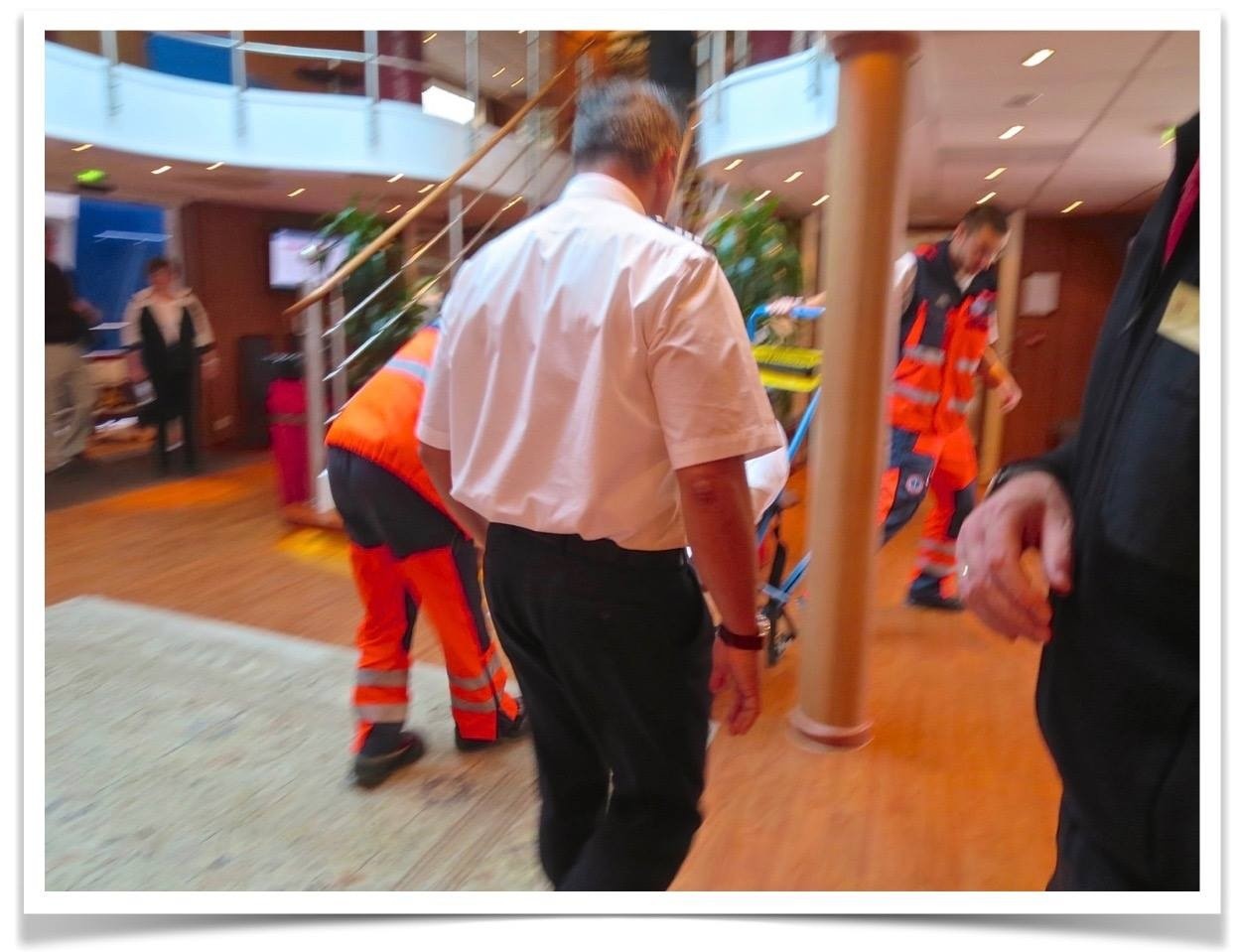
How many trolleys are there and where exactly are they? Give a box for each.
[688,300,829,666]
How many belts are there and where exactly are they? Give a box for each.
[512,523,686,574]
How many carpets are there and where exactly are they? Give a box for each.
[45,594,720,891]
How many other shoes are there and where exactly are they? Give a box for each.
[911,593,964,609]
[455,695,530,751]
[355,731,423,786]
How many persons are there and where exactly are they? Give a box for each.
[45,220,103,471]
[768,207,1021,610]
[414,80,783,892]
[323,316,532,785]
[122,256,217,473]
[956,112,1199,891]
[583,30,696,123]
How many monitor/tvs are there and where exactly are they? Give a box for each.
[268,225,353,291]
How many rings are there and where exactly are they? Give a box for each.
[958,564,968,580]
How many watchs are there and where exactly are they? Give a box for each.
[719,614,772,651]
